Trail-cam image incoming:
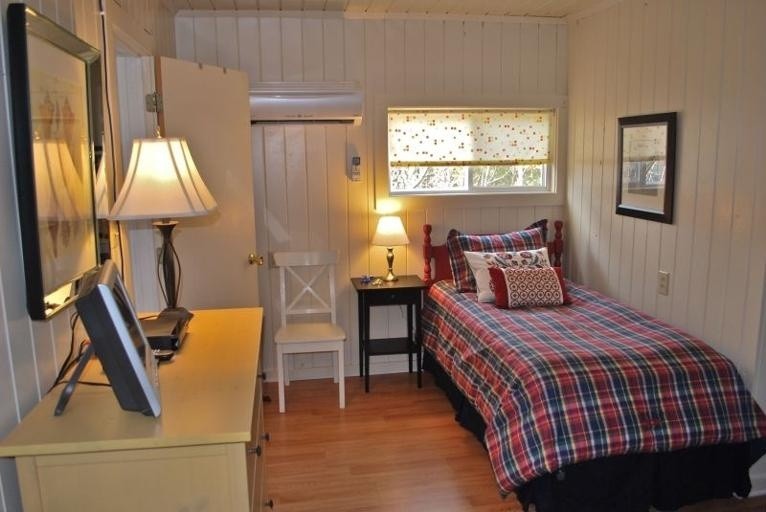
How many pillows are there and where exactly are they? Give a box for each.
[488,266,569,308]
[443,220,548,296]
[465,247,553,306]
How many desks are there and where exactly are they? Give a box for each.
[350,274,431,394]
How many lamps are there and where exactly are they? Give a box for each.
[105,125,219,337]
[372,216,411,282]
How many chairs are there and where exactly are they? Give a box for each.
[269,249,349,413]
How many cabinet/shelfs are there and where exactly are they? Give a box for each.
[0,305,268,512]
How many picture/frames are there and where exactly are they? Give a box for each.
[615,112,677,226]
[7,2,109,322]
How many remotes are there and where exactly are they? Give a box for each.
[151,348,174,360]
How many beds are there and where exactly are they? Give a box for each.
[421,218,766,511]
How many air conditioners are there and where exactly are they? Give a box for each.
[248,79,365,127]
